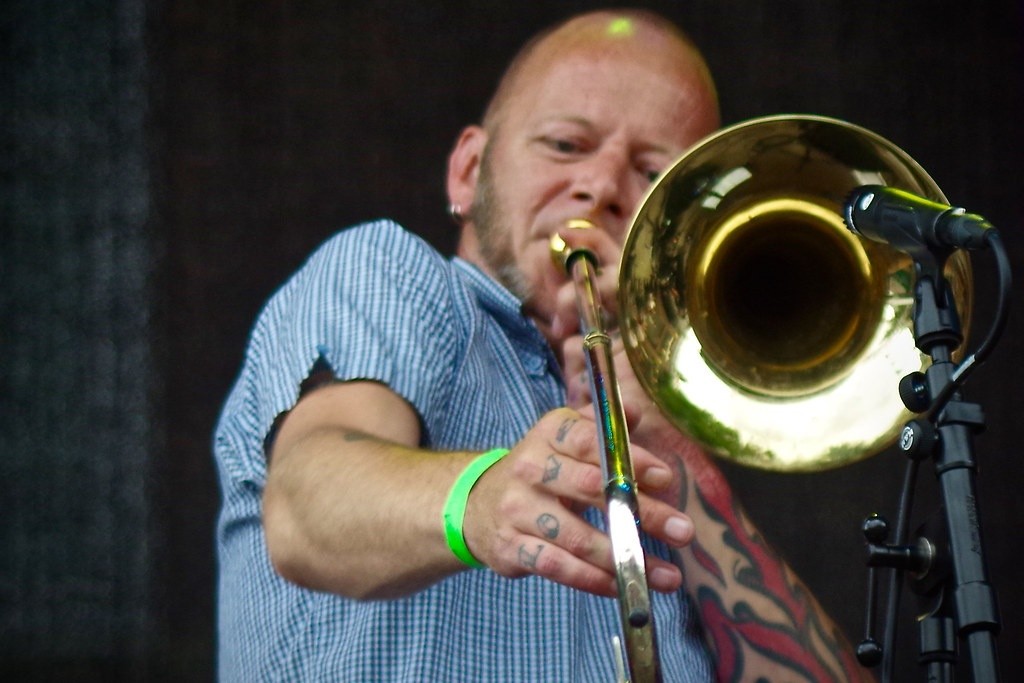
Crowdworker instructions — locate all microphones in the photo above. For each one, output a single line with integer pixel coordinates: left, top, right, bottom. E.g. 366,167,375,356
841,184,999,253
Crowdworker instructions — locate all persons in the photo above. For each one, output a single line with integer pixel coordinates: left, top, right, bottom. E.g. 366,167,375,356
211,9,724,682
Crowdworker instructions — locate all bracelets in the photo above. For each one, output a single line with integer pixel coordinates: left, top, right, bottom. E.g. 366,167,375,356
445,446,512,571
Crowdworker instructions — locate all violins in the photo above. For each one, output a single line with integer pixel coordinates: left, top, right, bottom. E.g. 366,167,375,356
548,114,979,682
